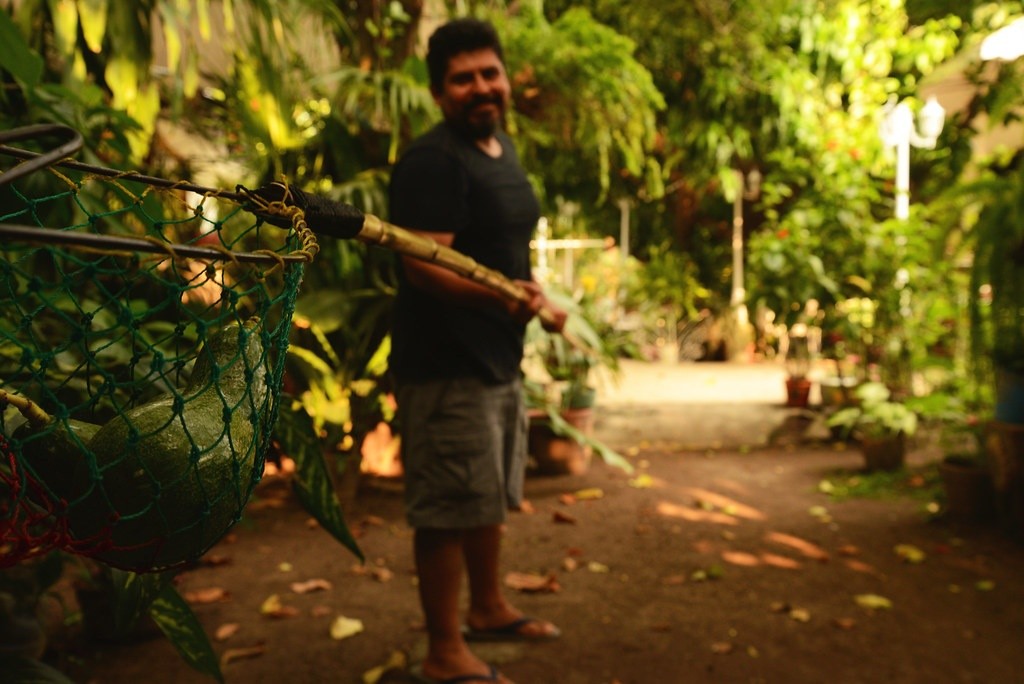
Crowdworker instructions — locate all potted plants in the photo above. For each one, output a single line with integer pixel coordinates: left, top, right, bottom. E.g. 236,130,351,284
810,384,915,472
522,268,647,480
916,199,1024,533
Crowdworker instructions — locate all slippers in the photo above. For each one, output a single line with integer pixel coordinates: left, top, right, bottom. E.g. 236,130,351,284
409,661,498,684
464,614,562,643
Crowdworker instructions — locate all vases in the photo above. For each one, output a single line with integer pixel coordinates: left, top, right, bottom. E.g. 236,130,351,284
76,574,169,645
785,375,814,410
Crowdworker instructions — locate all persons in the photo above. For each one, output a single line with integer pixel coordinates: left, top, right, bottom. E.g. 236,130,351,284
388,18,563,684
